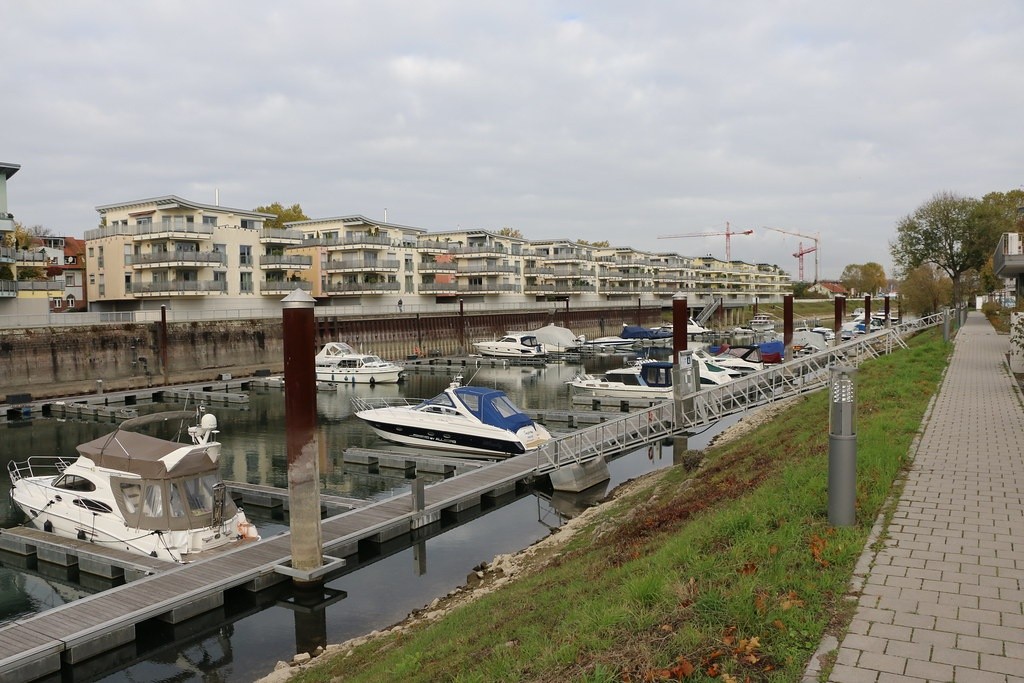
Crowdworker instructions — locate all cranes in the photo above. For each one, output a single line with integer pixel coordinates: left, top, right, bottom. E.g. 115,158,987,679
763,225,819,286
657,219,755,262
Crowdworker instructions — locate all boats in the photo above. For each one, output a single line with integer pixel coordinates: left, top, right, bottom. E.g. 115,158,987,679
470,332,551,358
562,347,786,408
565,308,901,358
353,381,554,457
315,342,404,384
8,407,263,564
